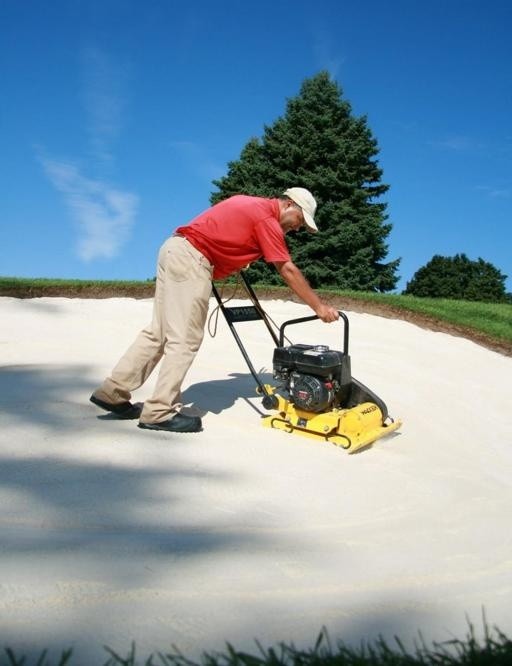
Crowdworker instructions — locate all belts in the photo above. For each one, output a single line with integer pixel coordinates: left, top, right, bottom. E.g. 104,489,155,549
172,233,186,239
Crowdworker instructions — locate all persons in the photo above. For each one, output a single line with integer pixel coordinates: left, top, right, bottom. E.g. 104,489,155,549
85,183,340,436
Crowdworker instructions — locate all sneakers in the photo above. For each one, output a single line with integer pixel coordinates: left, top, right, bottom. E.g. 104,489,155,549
138,413,200,432
90,395,142,418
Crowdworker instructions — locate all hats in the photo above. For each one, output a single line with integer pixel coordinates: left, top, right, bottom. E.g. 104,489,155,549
282,188,318,233
